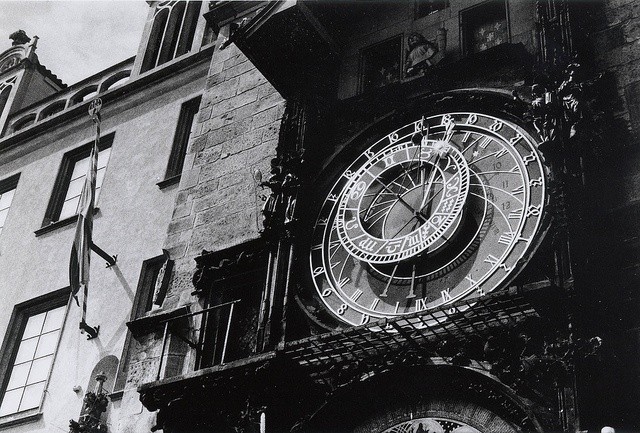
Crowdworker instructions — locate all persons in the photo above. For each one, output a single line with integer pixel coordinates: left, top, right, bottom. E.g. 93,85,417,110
406,31,437,75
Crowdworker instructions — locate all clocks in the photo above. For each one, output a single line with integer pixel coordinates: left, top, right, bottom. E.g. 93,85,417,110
290,359,543,432
286,86,560,344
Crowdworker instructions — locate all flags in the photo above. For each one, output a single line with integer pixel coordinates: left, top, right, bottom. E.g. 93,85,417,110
69,121,100,294
